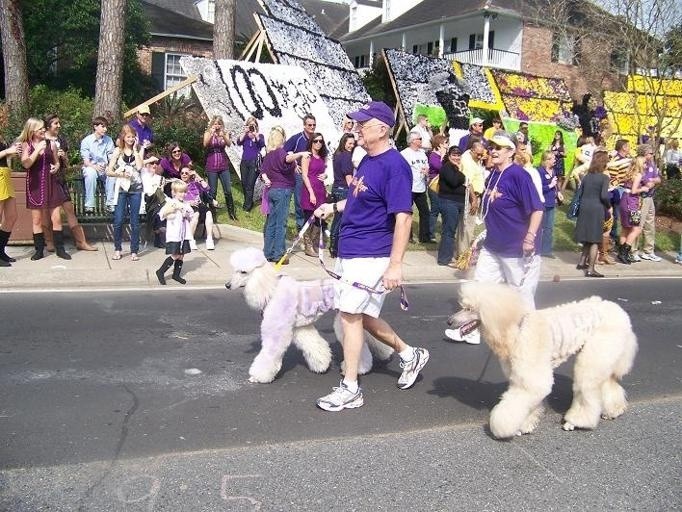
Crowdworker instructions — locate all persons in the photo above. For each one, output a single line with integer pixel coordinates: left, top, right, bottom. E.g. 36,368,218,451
80,116,115,214
436,73,473,146
571,93,612,146
47,113,98,252
0,105,23,267
12,117,71,260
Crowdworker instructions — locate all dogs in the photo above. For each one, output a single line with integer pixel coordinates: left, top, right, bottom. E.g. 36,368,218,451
444,278,641,439
224,245,397,384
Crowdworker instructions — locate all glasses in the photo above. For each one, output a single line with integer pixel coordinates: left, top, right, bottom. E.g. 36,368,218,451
487,146,511,152
356,122,384,130
312,140,323,144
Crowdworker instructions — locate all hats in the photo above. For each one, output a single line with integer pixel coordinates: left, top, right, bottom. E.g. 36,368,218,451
488,136,515,157
137,103,151,115
346,101,395,128
470,118,484,125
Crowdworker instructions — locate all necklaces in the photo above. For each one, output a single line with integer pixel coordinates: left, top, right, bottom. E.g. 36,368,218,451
26,139,46,207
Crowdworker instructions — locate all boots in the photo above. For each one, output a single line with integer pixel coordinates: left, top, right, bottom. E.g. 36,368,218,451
598,236,616,266
617,243,632,264
53,230,72,260
31,232,43,261
304,224,319,256
41,224,55,252
225,196,238,223
0,228,16,267
71,225,98,251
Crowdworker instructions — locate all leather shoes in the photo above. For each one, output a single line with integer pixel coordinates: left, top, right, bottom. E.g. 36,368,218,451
577,264,589,269
586,271,604,277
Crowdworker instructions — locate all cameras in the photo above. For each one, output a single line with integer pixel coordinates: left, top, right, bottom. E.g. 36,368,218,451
244,126,255,132
212,125,223,130
45,139,50,144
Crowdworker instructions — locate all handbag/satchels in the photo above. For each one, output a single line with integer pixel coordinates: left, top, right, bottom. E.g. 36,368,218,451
427,174,440,193
566,199,581,219
628,210,642,226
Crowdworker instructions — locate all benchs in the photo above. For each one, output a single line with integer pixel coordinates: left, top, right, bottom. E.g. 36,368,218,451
60,175,218,244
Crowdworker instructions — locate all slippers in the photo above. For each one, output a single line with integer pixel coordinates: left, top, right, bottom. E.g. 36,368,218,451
113,253,122,260
131,255,138,261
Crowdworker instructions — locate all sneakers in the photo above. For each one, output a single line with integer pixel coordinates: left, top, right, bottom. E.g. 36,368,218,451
628,252,641,262
642,252,662,261
206,239,215,250
157,270,166,285
315,379,364,412
190,240,197,250
107,206,115,214
172,275,185,284
444,327,481,346
85,207,94,213
396,347,430,390
